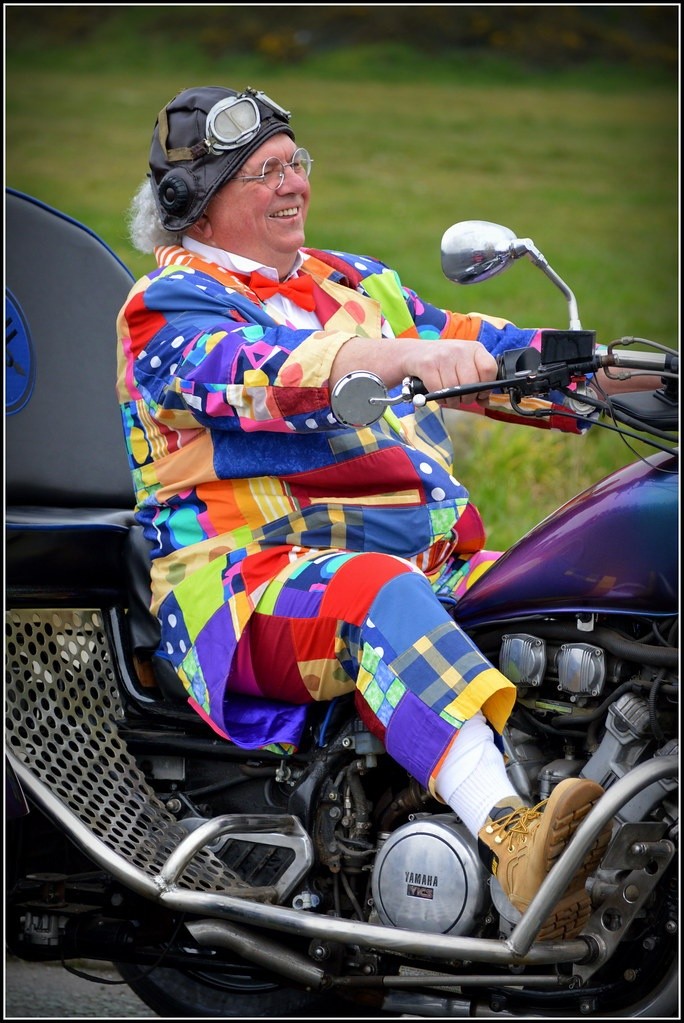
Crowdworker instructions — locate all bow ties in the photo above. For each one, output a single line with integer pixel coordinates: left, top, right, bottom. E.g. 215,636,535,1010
225,269,317,312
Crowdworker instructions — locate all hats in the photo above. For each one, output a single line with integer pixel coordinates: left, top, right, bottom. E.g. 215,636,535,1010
146,85,295,232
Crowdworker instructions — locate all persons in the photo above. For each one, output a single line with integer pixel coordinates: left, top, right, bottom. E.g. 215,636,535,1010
114,86,682,947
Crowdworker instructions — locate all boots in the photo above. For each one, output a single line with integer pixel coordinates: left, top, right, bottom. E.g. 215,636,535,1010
478,778,614,942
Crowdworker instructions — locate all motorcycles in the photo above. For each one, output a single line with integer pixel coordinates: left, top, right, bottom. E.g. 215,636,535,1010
3,181,679,1018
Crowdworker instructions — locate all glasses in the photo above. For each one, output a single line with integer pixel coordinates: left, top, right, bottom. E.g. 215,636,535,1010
205,91,291,150
229,147,314,190
